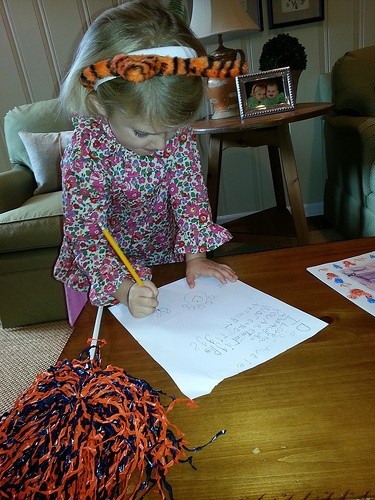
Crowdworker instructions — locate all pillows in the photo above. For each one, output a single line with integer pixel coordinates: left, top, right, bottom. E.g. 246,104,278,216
18,131,75,195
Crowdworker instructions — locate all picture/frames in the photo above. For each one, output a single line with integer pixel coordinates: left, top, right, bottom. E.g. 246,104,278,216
236,66,295,120
266,0,324,30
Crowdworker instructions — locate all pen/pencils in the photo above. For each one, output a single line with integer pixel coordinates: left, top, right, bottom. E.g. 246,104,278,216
95,219,146,287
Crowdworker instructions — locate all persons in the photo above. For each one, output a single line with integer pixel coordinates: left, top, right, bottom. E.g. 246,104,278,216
53,1,238,326
246,83,287,109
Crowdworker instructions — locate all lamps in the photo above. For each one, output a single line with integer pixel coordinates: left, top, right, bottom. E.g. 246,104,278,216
188,0,262,121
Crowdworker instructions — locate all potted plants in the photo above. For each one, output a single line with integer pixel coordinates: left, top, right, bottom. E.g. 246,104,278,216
258,33,308,105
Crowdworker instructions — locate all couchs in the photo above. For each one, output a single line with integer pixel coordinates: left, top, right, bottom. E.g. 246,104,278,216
0,97,72,330
323,46,375,237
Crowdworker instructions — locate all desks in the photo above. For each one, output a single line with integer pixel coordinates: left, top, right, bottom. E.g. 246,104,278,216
57,236,375,500
192,100,336,257
